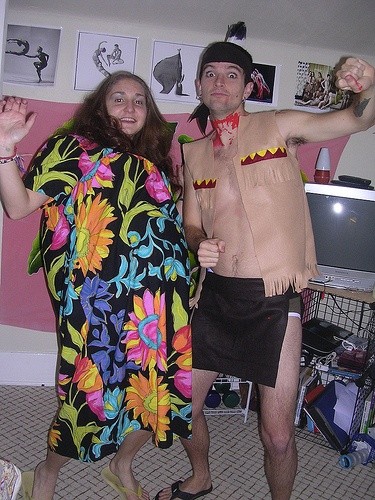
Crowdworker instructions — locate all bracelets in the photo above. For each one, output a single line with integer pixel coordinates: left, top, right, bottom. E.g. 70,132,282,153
0,145,27,176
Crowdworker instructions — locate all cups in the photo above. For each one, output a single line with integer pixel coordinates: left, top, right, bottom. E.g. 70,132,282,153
315,148,331,171
314,170,330,184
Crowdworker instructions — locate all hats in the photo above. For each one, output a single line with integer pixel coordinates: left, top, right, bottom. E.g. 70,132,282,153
201,42,254,78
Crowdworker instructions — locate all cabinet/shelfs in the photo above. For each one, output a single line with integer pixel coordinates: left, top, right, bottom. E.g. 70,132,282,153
203,282,375,458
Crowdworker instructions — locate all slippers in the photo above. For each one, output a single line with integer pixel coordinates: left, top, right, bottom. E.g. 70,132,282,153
155,480,213,500
21,470,35,500
101,466,143,500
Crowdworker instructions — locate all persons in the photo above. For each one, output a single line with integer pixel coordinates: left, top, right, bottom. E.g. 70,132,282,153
25,46,49,82
295,71,351,110
107,44,124,67
251,68,270,100
0,71,193,500
92,41,110,78
5,39,30,56
154,22,375,500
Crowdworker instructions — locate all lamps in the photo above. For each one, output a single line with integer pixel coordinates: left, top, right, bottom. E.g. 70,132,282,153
314,147,331,184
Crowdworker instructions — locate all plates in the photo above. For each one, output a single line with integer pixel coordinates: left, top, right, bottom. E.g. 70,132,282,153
338,175,371,186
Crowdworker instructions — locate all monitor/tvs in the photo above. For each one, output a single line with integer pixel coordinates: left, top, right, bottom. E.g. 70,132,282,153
304,182,375,295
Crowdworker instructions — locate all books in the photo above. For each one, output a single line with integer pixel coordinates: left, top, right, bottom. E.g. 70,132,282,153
294,367,375,453
